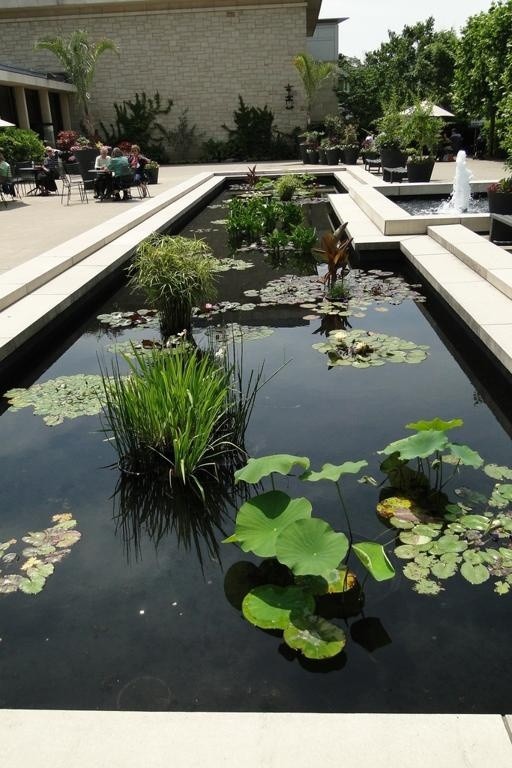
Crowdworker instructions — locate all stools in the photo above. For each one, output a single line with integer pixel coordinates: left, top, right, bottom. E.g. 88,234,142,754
383,167,408,183
489,210,512,246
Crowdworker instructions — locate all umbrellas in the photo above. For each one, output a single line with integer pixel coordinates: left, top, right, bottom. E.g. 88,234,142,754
0,119,16,126
396,99,457,117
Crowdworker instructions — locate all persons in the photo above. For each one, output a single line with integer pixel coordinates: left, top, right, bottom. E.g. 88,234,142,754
32,146,59,196
361,123,488,162
104,147,136,201
127,144,151,197
93,147,112,198
0,147,12,192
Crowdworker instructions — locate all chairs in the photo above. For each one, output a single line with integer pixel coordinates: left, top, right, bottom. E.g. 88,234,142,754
0,162,150,208
365,147,384,174
64,150,82,174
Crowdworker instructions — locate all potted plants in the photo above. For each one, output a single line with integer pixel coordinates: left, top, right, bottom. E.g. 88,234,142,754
298,120,358,165
359,147,381,164
487,174,512,213
144,161,160,184
368,85,418,171
70,136,99,180
399,88,447,182
